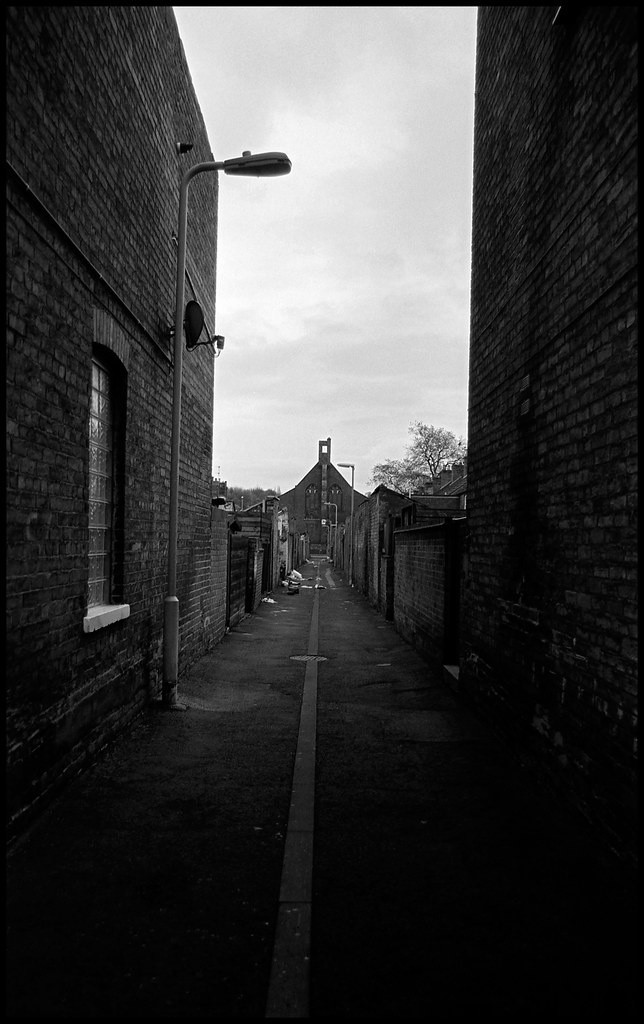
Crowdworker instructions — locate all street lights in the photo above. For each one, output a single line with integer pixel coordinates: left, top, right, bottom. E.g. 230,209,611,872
160,150,293,711
338,462,355,586
324,502,338,567
